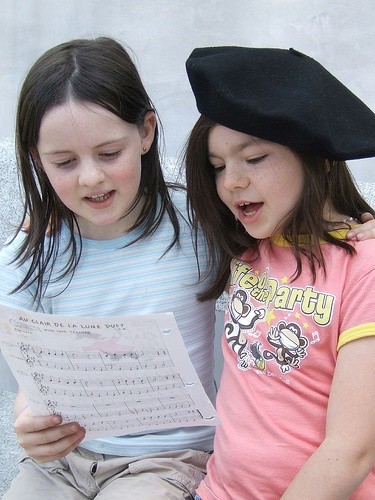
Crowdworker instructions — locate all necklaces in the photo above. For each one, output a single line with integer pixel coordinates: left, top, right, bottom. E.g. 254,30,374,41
321,213,357,230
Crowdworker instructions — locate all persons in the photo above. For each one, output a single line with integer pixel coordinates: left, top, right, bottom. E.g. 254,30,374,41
18,45,375,500
0,37,375,499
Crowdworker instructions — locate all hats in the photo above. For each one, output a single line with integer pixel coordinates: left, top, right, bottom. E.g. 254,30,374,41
185,47,374,161
